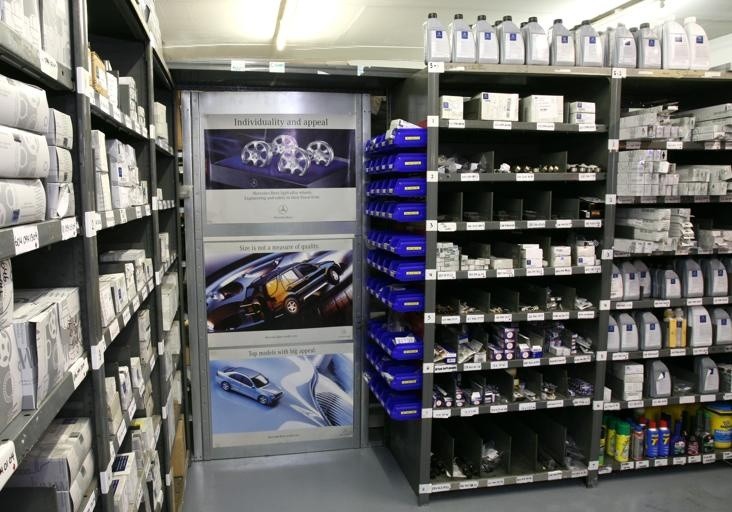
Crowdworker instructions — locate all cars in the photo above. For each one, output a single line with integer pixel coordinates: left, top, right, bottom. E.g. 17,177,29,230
217,365,285,404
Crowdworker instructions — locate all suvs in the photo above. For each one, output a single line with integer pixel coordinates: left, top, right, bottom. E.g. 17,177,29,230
240,261,342,321
206,251,334,332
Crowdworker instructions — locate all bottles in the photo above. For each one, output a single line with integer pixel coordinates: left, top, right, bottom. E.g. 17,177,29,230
599,410,717,466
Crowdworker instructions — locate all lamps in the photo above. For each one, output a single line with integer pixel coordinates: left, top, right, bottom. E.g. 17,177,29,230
272,0,296,50
567,0,663,30
